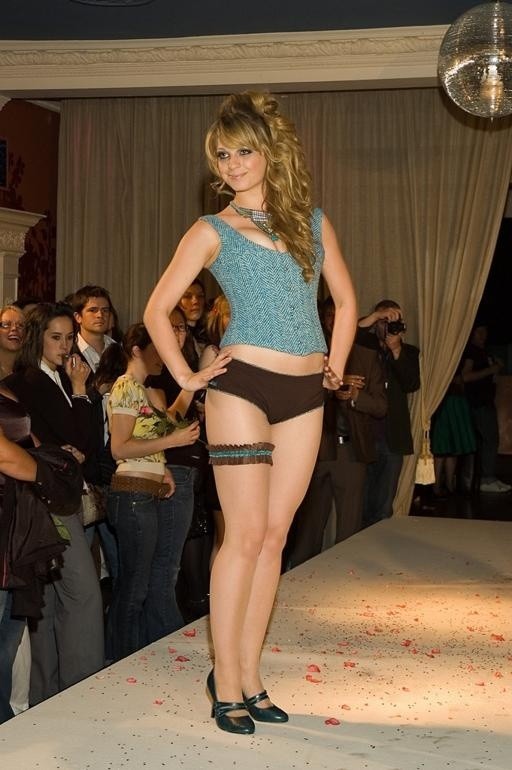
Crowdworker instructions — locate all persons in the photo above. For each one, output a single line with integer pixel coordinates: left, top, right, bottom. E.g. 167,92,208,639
142,92,360,735
100,323,202,661
415,322,505,512
286,294,421,570
1,279,230,724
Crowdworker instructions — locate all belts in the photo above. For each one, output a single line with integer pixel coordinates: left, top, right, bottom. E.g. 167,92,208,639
335,434,350,445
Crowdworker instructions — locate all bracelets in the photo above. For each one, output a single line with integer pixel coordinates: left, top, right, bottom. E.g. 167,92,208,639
322,356,344,386
72,394,92,403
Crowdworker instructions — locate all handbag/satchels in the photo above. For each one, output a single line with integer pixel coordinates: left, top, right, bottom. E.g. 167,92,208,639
80,486,108,528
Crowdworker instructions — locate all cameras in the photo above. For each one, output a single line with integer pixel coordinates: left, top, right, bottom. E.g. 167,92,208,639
384,314,407,336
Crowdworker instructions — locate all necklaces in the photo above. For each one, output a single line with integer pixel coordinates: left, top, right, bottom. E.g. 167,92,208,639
229,197,283,241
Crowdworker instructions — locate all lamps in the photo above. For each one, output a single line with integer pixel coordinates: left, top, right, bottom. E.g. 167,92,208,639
435,2,512,125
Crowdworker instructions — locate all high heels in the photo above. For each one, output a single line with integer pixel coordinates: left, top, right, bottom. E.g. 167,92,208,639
242,686,289,723
207,666,254,733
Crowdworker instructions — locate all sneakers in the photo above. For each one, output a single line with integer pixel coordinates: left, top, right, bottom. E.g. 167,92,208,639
480,478,511,492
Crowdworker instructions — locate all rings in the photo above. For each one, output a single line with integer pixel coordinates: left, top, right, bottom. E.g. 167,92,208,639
65,447,73,453
82,364,85,366
